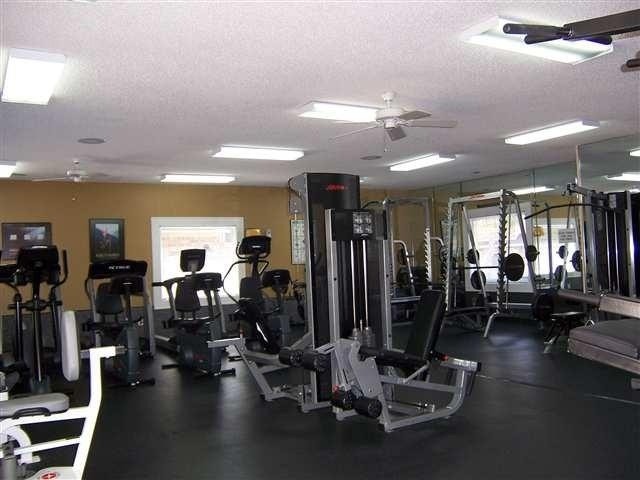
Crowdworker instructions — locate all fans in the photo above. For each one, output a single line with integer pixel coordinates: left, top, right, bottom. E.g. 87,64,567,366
330,85,433,149
32,158,123,187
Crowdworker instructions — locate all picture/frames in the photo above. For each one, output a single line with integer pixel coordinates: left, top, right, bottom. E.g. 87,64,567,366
1,222,52,261
88,217,126,263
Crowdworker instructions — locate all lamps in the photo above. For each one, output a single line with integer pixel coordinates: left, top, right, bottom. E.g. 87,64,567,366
503,118,603,147
388,152,458,173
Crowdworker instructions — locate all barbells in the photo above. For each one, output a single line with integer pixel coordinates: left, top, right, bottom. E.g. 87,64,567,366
441,254,524,281
